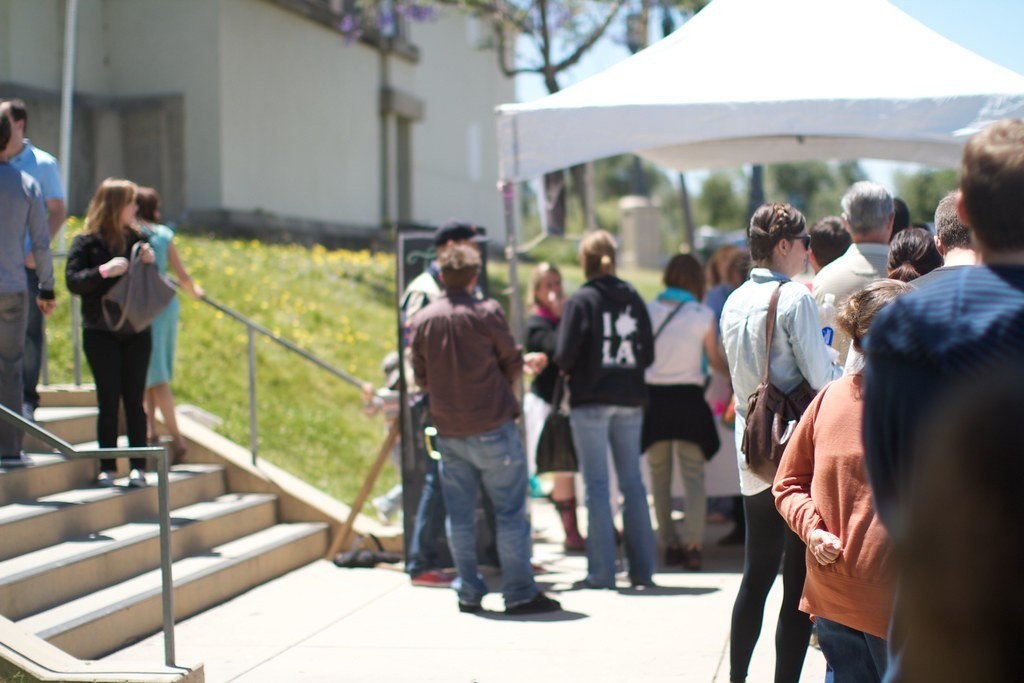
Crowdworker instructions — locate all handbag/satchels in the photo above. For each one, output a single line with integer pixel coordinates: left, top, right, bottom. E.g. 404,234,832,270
102,240,177,332
536,374,580,476
741,379,819,483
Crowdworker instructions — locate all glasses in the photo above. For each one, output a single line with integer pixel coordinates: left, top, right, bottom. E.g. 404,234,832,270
787,235,812,252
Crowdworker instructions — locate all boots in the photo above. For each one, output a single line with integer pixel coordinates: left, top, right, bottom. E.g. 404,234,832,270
548,494,586,551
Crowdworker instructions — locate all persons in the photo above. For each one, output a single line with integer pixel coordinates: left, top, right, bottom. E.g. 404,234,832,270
0,97,66,467
131,187,204,465
400,120,1024,682
65,177,156,489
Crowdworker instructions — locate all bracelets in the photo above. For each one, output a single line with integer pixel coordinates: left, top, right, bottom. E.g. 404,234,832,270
98,264,107,278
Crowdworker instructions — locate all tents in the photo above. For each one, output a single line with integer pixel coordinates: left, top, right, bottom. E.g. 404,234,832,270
495,1,1024,459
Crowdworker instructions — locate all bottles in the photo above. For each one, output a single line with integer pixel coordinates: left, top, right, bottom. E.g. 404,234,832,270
818,293,838,350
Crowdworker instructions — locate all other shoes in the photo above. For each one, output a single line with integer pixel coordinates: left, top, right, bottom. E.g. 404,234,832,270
0,455,34,466
658,542,703,571
22,405,35,422
128,469,147,487
573,577,592,589
412,569,458,587
717,529,745,547
458,601,481,612
96,472,113,486
505,590,561,615
